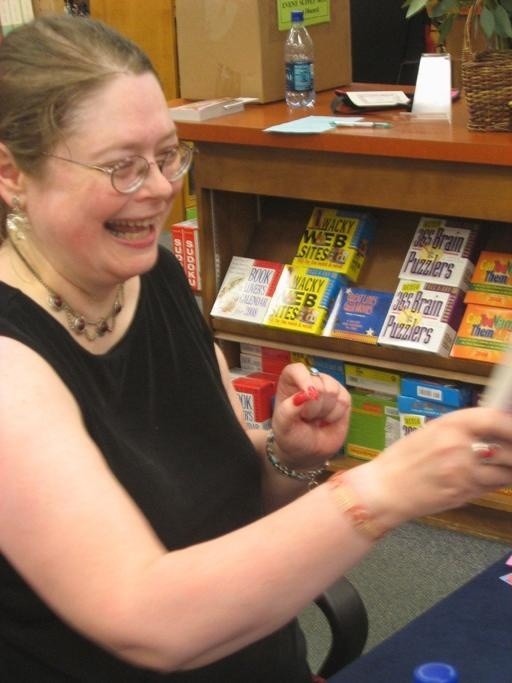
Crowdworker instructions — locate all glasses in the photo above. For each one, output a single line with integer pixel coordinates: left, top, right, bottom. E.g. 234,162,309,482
37,137,201,196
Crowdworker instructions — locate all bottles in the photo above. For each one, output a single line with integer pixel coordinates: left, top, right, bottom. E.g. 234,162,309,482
281,9,317,107
412,662,460,682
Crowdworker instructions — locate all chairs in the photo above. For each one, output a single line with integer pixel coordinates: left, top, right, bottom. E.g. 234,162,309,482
310,574,370,681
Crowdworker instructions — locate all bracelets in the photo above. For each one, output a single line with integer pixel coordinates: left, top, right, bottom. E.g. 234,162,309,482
263,432,329,487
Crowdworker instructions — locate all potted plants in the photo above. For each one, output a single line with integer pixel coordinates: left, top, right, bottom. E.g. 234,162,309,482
398,0,512,133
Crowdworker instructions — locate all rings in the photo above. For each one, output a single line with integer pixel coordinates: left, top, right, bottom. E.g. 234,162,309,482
472,439,501,464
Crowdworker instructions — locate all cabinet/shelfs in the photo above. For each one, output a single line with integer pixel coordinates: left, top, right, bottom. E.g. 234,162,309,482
164,98,512,546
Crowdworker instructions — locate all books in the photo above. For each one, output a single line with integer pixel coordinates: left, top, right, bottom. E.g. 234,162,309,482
173,96,245,122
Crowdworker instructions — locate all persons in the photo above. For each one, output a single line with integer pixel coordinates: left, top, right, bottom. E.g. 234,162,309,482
0,15,509,683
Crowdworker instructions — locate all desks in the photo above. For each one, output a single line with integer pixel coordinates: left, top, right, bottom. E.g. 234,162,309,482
327,548,512,682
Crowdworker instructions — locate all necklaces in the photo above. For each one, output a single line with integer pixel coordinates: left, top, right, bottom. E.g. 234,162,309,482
7,229,131,342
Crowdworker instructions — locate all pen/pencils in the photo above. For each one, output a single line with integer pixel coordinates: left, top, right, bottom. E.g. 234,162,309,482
328,120,392,128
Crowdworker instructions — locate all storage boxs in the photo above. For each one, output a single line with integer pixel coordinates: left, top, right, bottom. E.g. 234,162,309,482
173,0,354,107
